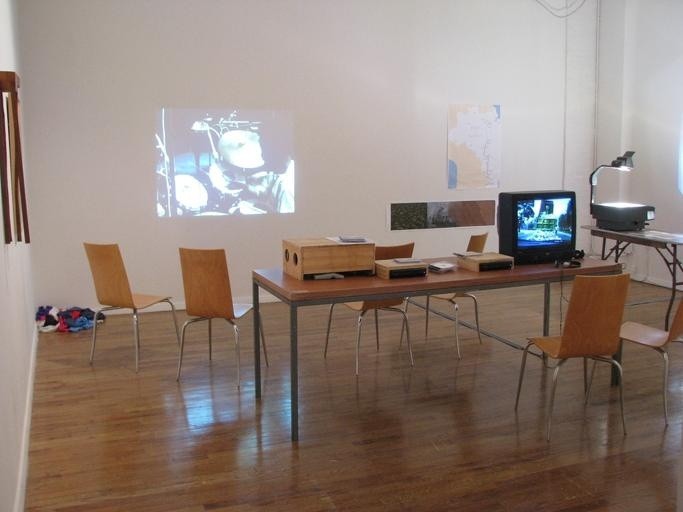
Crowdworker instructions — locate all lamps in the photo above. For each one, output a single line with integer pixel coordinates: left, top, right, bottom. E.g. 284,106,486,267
398,233,489,360
514,272,631,443
175,247,269,390
583,292,683,427
325,242,416,376
83,242,181,374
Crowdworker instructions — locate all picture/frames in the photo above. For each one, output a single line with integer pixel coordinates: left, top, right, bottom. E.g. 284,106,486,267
252,255,622,443
586,227,683,331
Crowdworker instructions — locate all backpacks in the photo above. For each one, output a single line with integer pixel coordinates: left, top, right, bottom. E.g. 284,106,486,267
208,130,264,195
174,174,208,212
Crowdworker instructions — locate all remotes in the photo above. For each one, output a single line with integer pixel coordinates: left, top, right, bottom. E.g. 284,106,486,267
563,261,580,267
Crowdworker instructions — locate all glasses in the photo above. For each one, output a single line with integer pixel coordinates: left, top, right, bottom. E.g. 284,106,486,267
589,150,655,232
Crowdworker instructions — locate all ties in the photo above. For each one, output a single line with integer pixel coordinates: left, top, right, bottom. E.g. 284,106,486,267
499,190,576,264
